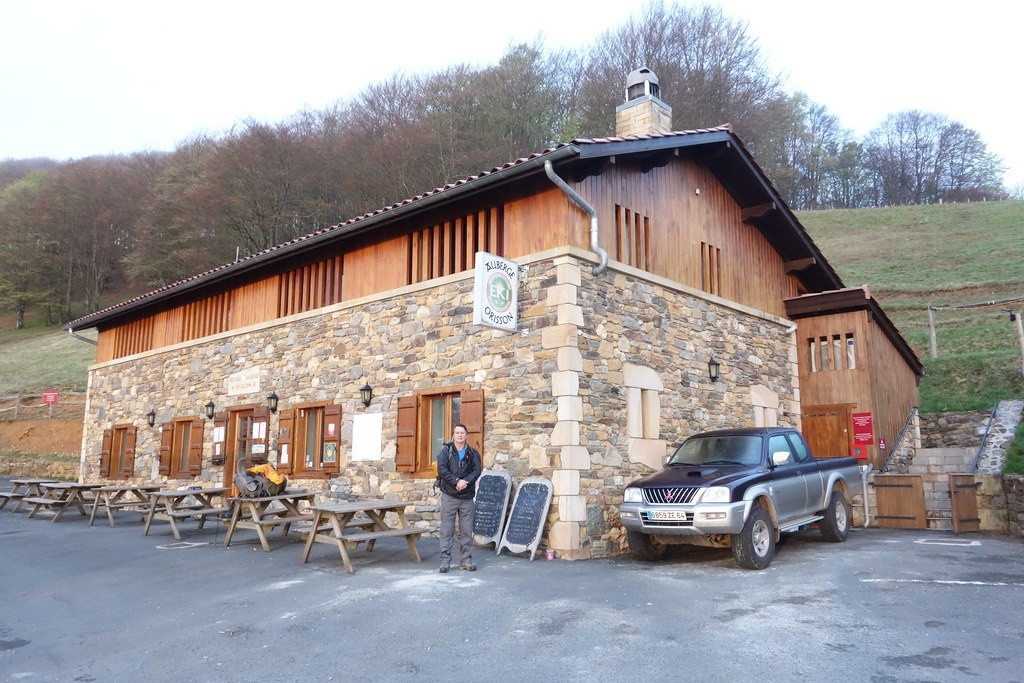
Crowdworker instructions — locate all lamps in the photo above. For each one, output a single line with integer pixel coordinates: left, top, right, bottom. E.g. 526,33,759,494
267,390,278,413
205,399,215,419
709,357,720,382
359,382,372,407
146,409,155,426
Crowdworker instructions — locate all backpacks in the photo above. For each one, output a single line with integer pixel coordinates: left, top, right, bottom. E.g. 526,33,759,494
434,440,454,487
231,456,287,498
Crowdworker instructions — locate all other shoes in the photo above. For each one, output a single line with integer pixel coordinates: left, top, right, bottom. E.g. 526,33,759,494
439,562,450,572
460,558,476,571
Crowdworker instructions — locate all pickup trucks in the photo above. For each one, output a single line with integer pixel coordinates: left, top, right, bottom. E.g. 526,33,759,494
619,425,862,571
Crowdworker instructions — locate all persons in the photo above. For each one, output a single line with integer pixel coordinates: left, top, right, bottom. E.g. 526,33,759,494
437,423,481,572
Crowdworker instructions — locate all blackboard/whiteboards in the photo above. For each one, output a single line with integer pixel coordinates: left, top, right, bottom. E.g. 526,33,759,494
472,474,507,537
505,483,549,546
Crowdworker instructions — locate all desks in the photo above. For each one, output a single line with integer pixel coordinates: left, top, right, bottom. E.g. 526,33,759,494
89,485,167,527
141,488,231,539
299,499,421,574
28,483,107,522
222,491,326,551
0,479,62,513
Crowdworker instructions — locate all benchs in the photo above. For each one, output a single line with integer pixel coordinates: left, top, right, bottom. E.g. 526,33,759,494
168,507,230,519
253,515,314,525
291,521,374,535
0,492,167,509
136,505,204,514
218,511,284,522
337,526,429,542
